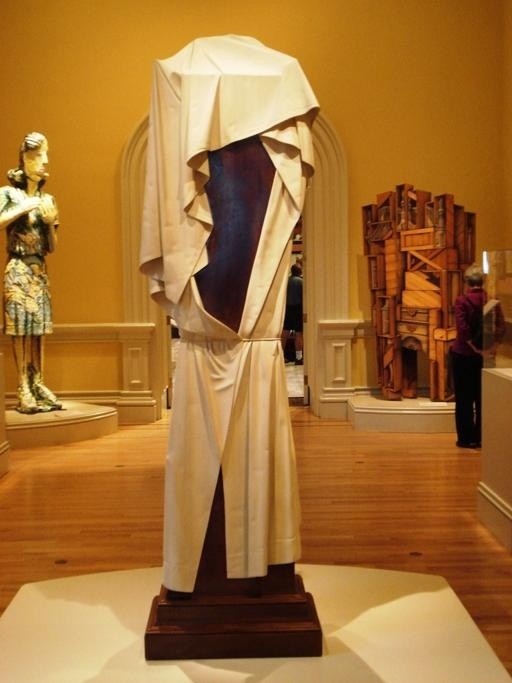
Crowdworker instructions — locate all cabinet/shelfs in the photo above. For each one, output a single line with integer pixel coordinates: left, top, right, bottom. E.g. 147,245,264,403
473,249,511,546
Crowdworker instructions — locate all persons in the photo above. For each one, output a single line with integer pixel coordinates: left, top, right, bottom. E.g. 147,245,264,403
1,128,64,418
445,266,507,451
286,261,304,305
